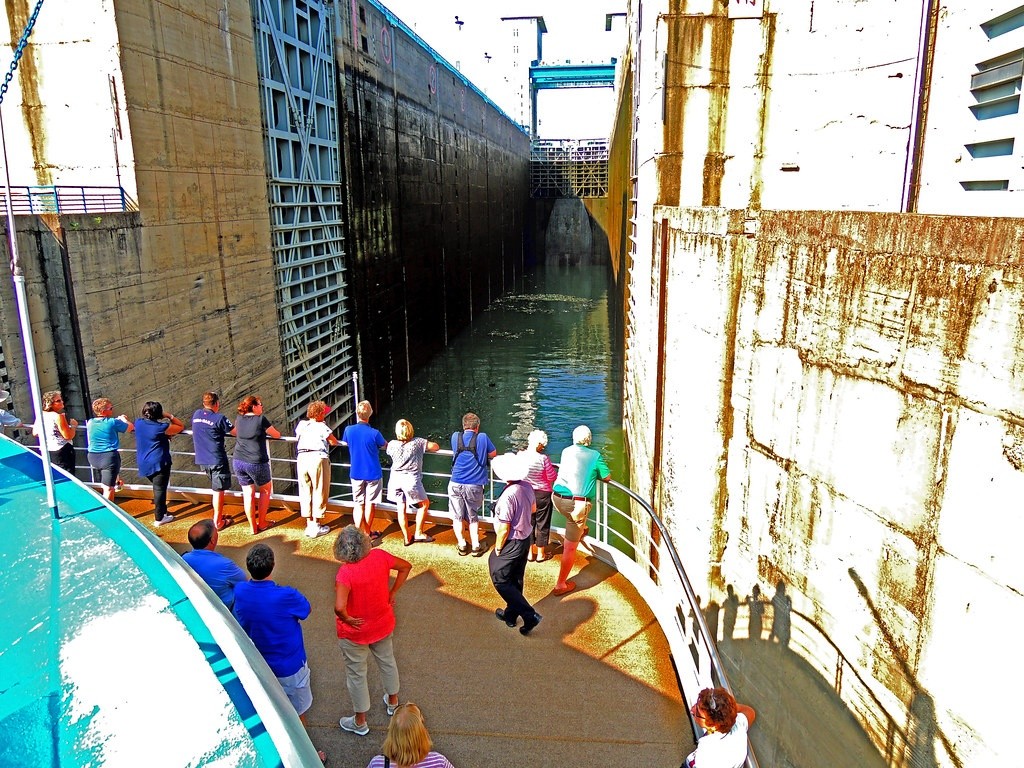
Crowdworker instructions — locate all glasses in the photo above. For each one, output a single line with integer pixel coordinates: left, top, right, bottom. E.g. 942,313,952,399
105,407,113,411
54,399,61,403
694,702,706,720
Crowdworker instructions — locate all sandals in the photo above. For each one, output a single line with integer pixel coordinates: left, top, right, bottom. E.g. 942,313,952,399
458,541,472,556
471,542,488,557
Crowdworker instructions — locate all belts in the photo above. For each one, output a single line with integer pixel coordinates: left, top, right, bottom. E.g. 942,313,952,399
554,492,591,501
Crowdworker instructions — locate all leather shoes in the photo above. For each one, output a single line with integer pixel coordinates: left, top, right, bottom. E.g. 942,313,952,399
496,608,518,627
520,613,542,635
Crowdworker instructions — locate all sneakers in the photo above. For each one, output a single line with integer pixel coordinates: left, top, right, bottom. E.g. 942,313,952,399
382,694,398,715
155,514,173,526
304,526,311,535
340,716,370,735
310,522,330,538
114,479,124,493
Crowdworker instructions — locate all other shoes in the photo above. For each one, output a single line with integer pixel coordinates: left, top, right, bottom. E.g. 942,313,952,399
554,582,576,596
580,525,590,540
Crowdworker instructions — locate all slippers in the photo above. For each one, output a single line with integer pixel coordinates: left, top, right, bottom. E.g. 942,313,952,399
527,558,535,562
414,534,436,542
405,535,414,546
261,521,275,530
369,532,382,540
537,553,553,562
219,519,234,531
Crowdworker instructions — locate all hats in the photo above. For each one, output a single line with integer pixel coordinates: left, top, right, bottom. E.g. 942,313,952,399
491,453,528,481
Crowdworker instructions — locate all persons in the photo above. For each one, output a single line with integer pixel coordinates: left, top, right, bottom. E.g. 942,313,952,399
342,401,388,540
295,401,338,538
550,425,611,596
487,453,543,636
386,419,439,546
334,525,412,736
180,519,250,611
86,398,135,503
517,430,558,563
134,401,185,527
367,701,455,768
681,688,755,768
231,544,327,764
230,396,280,535
447,413,496,558
33,391,78,475
0,390,23,434
191,392,236,532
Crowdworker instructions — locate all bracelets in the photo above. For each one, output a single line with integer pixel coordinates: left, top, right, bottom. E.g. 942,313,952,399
71,425,77,428
495,548,501,551
168,414,173,420
